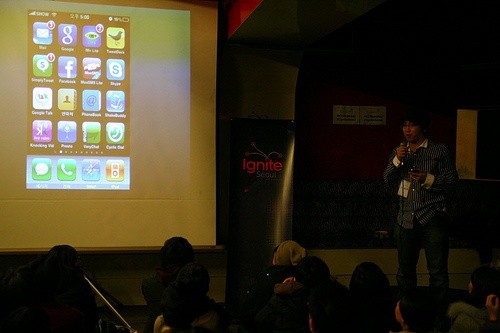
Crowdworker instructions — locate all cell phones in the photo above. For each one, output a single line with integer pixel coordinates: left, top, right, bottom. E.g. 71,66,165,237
409,169,420,173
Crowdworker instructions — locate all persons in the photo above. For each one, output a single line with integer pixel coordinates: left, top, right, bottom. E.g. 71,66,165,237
0,237,500,333
383,110,459,326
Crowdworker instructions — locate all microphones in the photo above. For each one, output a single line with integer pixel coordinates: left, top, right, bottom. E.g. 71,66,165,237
400,142,406,166
